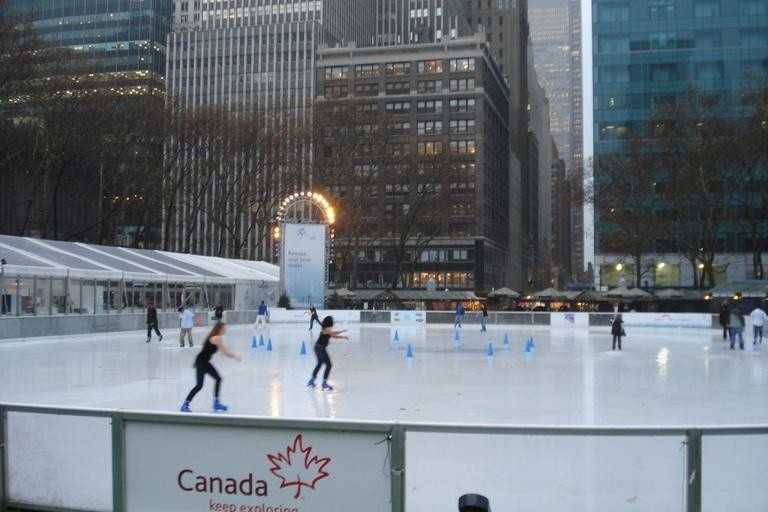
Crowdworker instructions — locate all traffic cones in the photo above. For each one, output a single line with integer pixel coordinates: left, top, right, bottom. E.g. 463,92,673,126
525,340,531,353
405,343,414,358
258,333,264,345
266,338,273,351
486,342,494,356
251,336,257,348
502,332,511,344
455,327,460,341
299,340,309,355
529,336,535,348
393,329,399,341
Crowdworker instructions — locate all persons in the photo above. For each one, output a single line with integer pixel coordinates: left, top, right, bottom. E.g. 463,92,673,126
751,305,768,345
253,299,270,329
727,309,746,349
304,301,322,331
145,300,163,342
181,321,241,411
452,303,466,328
211,303,222,321
308,315,348,389
178,305,193,347
475,304,488,331
720,304,731,338
612,314,625,351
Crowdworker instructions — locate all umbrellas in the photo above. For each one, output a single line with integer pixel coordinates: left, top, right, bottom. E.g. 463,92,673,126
488,286,686,311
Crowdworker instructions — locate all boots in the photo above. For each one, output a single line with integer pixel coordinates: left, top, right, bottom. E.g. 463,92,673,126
321,380,332,387
181,400,192,412
308,379,315,385
213,399,226,409
